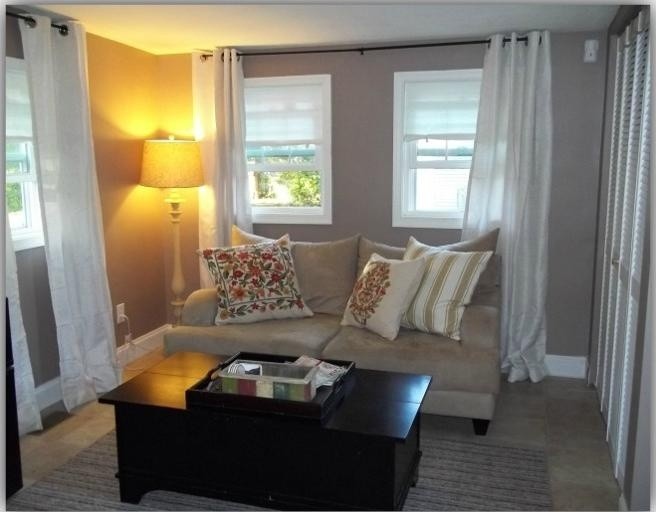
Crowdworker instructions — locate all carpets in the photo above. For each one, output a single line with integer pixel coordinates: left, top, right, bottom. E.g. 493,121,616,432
5,429,557,512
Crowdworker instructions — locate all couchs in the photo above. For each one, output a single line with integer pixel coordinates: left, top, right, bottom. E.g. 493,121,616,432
163,225,509,435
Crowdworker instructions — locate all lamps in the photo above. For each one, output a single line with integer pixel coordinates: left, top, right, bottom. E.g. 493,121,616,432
141,139,205,327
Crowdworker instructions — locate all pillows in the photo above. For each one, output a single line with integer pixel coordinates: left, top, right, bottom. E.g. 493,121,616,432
196,231,493,342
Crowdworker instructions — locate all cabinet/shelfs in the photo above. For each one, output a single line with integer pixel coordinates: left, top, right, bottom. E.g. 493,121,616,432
589,4,656,484
5,296,25,496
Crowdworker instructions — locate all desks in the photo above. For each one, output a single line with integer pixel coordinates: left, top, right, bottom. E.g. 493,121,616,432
99,348,434,511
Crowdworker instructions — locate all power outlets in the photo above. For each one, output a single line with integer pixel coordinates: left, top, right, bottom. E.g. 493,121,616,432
114,302,127,323
